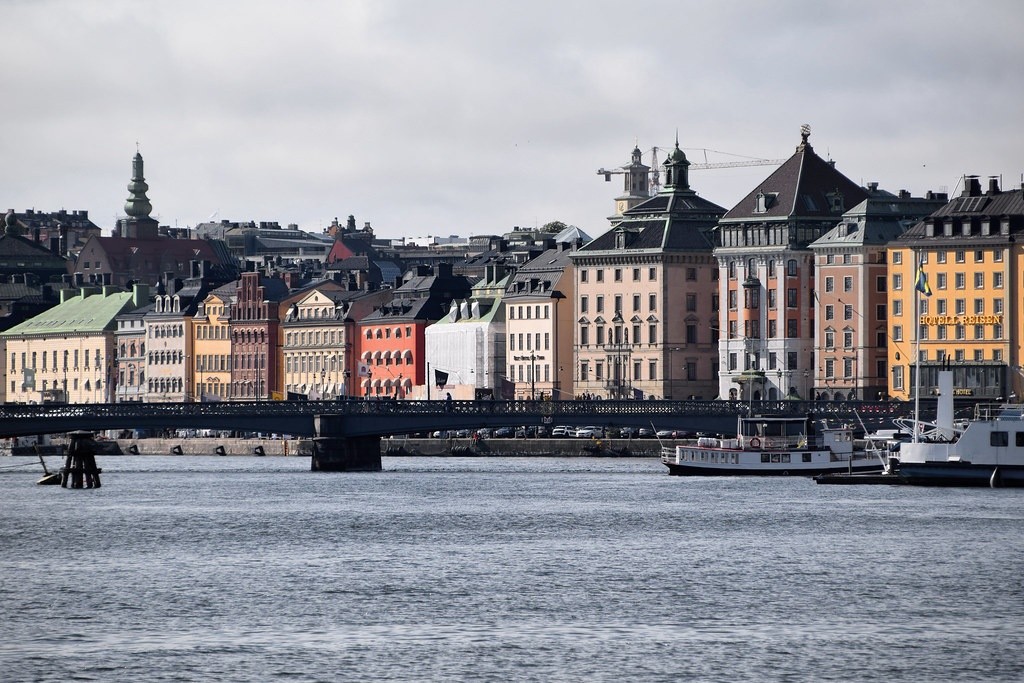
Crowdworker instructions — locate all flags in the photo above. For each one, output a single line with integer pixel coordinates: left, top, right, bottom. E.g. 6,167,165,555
23,368,35,389
272,390,284,401
202,392,221,402
360,326,412,387
358,361,369,376
914,266,932,297
435,369,448,387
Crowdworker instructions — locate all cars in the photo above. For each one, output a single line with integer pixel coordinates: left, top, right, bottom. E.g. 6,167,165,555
156,427,691,439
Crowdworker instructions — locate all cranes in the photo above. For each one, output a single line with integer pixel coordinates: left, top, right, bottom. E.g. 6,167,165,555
592,144,786,197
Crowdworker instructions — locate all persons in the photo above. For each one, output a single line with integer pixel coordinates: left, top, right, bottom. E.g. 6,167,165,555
582,393,591,401
815,392,821,412
446,392,452,411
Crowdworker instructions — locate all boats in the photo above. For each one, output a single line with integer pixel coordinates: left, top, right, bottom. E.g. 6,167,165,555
653,415,896,475
886,402,1024,483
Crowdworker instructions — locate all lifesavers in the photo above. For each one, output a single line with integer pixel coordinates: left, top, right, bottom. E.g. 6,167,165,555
749,437,761,448
